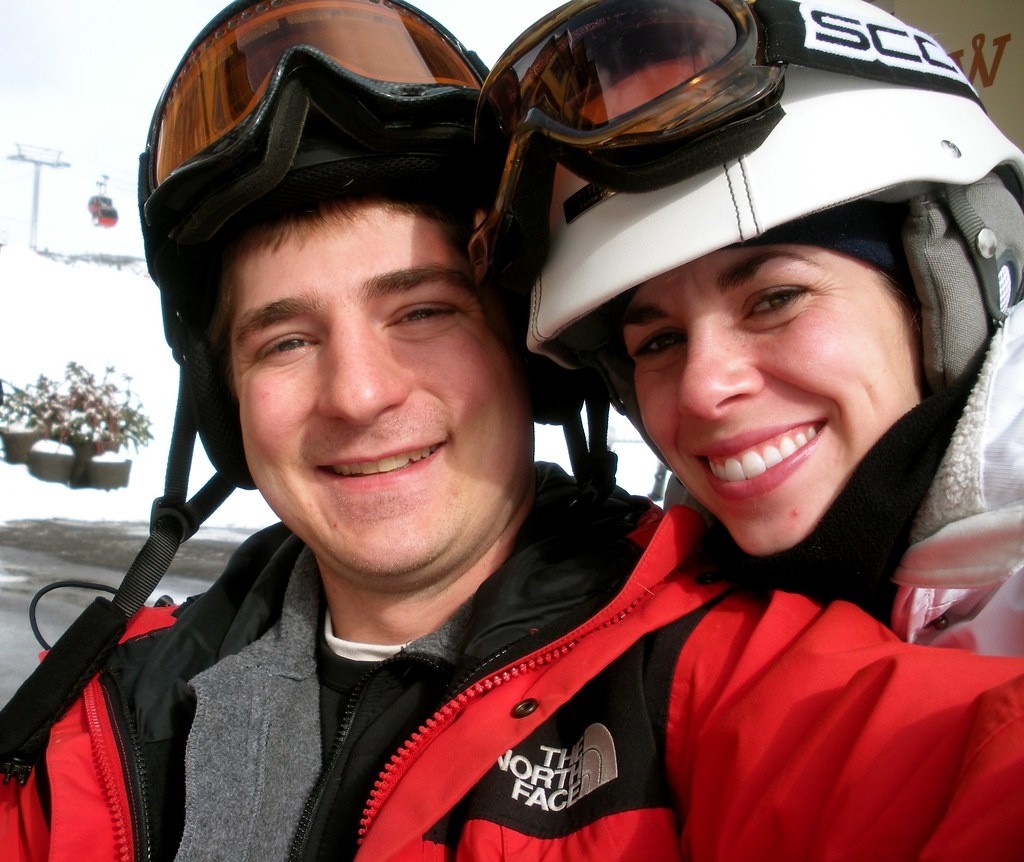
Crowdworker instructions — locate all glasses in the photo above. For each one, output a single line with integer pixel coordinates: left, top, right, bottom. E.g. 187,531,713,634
468,0,987,333
134,0,493,345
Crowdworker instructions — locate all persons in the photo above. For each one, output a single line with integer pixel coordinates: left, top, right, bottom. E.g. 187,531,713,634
0,0,1024,862
471,0,1024,733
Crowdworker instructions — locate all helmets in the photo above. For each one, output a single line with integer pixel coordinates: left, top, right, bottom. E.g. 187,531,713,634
524,0,1024,375
